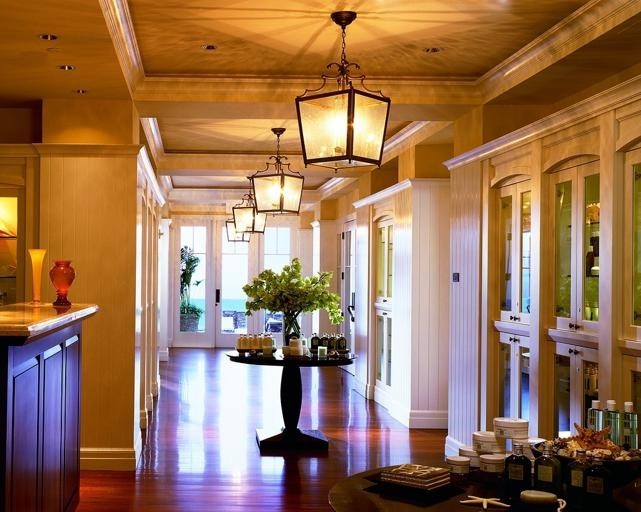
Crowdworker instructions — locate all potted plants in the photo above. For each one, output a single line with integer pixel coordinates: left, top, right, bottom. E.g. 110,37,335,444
180,246,204,331
242,257,344,345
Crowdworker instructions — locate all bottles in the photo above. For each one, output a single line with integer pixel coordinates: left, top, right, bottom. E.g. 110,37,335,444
299,331,346,359
585,202,599,258
584,300,591,320
584,245,594,275
504,399,638,512
235,331,275,356
583,364,599,398
592,302,598,321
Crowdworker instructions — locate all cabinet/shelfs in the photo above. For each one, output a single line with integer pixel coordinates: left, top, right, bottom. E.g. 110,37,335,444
442,75,641,464
352,178,450,429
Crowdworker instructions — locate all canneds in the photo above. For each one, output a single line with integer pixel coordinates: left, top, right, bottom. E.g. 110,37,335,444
447,417,546,486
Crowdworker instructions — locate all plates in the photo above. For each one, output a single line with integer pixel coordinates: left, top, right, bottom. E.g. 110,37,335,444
360,472,471,498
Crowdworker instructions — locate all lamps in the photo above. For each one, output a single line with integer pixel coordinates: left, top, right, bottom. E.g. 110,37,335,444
226,218,251,243
233,177,267,235
296,11,390,173
251,128,304,217
